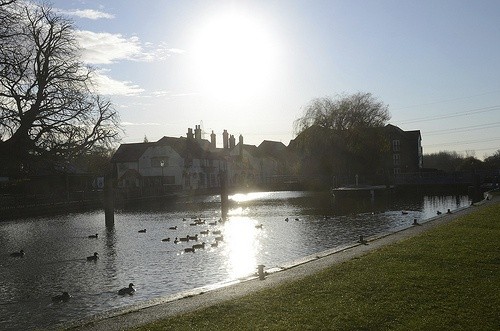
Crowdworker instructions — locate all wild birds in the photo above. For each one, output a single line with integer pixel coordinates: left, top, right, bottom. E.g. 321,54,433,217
11,209,443,303
